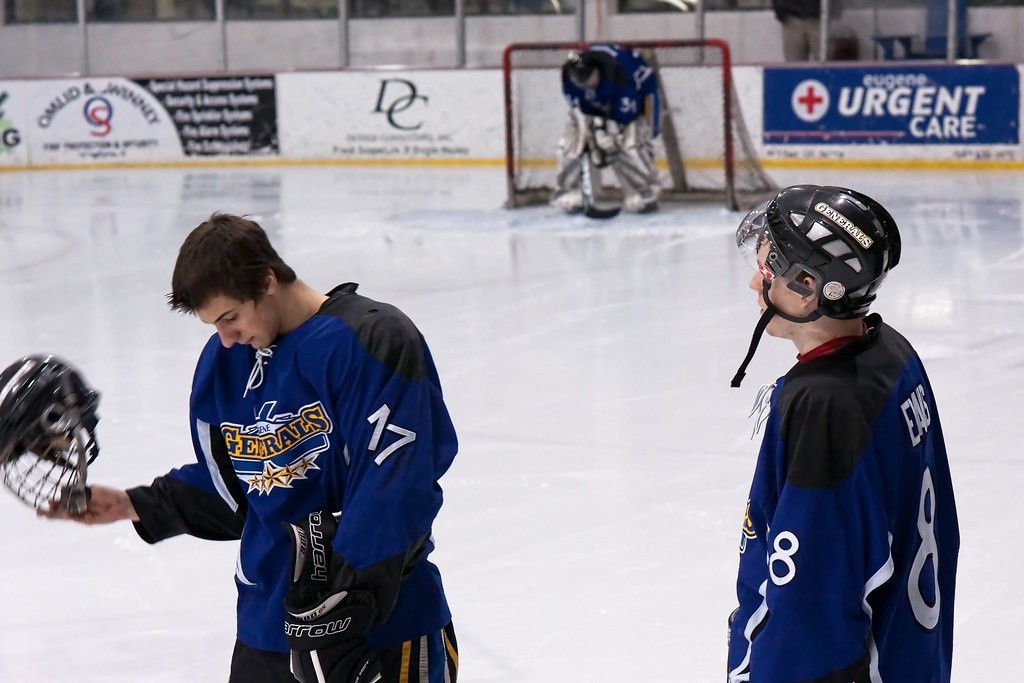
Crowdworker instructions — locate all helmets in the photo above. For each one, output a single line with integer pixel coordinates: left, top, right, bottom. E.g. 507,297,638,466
736,184,901,320
567,50,598,85
0,353,102,514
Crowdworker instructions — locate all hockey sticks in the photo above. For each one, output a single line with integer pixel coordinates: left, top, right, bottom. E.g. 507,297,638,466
582,146,621,219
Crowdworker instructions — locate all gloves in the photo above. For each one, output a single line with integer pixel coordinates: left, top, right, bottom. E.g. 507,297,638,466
281,509,382,683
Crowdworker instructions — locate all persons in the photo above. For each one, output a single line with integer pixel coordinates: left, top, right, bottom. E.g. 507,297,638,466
730,183,961,682
37,214,461,683
553,43,665,219
